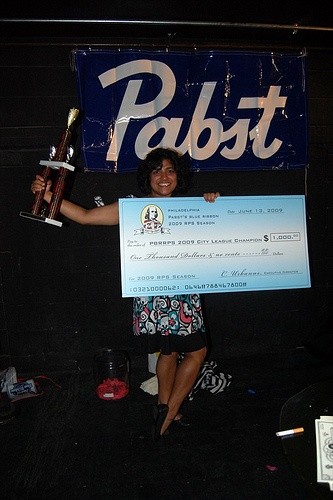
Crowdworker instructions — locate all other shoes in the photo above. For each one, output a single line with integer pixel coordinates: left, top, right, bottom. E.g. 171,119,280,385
155,404,191,427
152,435,171,463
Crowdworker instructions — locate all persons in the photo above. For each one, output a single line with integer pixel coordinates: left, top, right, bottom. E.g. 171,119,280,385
31,147,222,441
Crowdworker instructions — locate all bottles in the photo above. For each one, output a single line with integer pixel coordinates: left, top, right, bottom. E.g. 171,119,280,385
92,344,129,400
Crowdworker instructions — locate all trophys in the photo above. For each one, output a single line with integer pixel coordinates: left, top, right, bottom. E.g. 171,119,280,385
20,107,78,228
